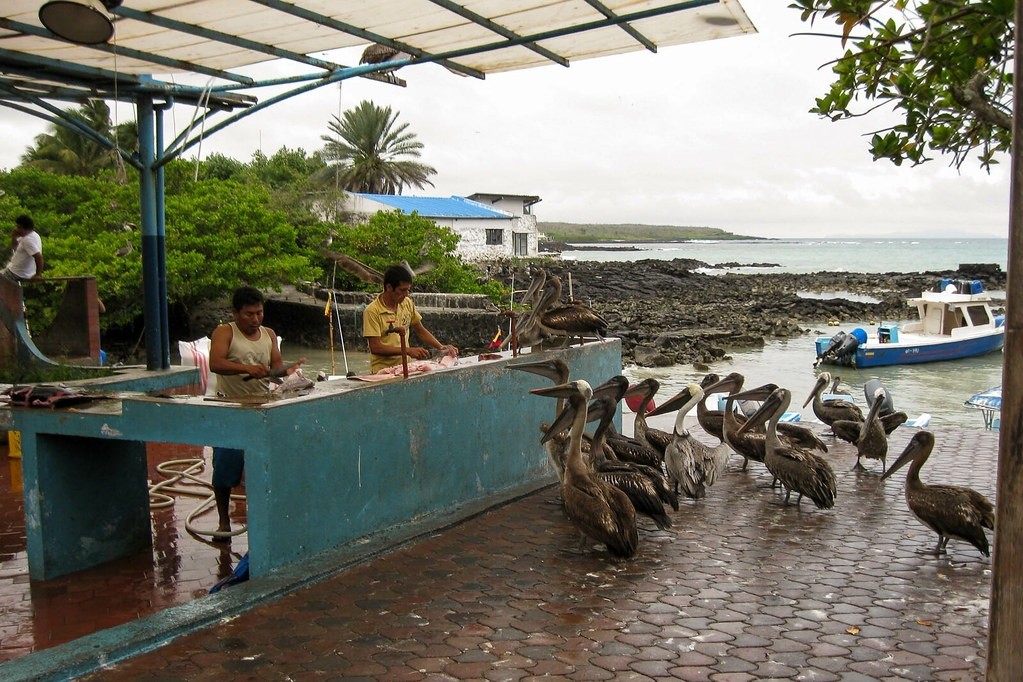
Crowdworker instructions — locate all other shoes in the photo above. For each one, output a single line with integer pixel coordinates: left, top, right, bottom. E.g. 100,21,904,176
212,528,231,541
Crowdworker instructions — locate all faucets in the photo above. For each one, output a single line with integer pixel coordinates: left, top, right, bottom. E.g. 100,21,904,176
384,320,396,336
496,304,506,319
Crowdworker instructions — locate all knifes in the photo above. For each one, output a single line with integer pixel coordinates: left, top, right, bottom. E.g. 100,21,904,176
429,348,440,356
243,366,292,381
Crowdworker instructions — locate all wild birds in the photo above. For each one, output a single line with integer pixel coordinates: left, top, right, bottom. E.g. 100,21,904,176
501,358,910,561
319,229,436,288
879,430,996,558
497,269,609,355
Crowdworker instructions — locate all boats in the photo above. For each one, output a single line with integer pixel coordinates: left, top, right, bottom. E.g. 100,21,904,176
813,278,1006,371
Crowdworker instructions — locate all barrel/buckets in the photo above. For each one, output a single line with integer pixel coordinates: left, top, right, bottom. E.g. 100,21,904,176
995,313,1006,327
940,278,983,294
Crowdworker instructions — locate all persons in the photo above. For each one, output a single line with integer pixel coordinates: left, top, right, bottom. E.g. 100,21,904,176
209,288,305,542
362,265,458,376
0,215,43,312
